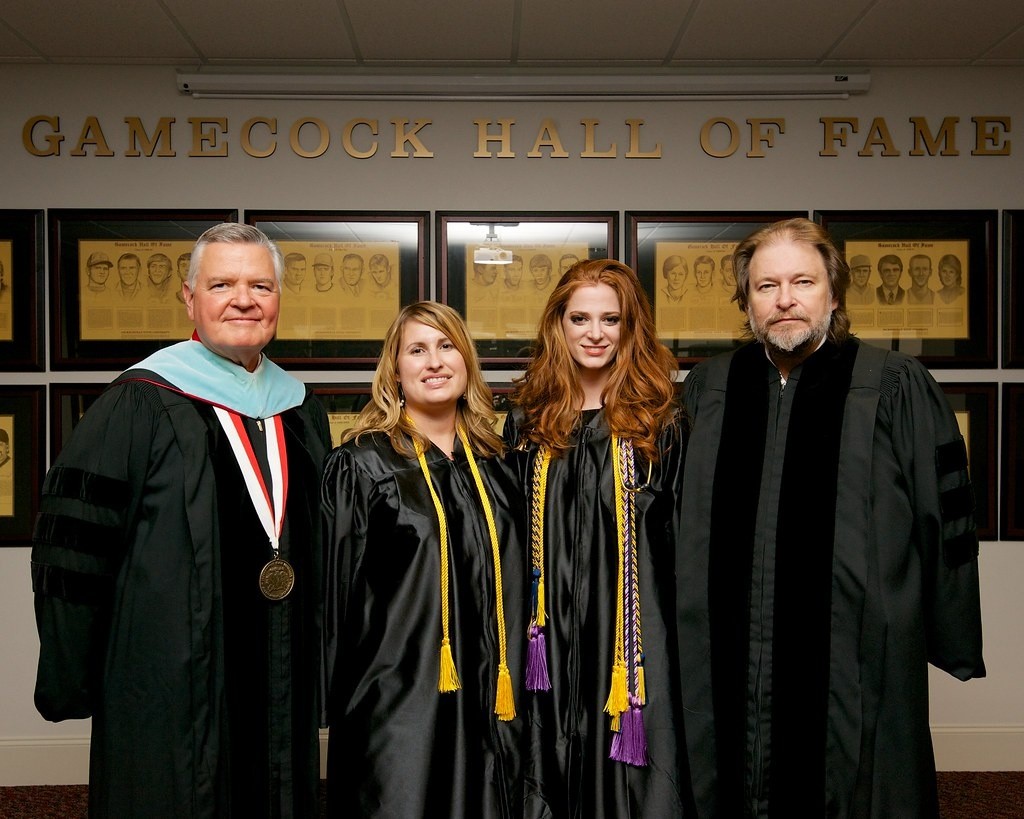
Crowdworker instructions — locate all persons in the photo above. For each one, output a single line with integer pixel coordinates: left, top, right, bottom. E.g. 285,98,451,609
501,257,702,819
663,217,992,819
33,218,339,819
311,298,535,818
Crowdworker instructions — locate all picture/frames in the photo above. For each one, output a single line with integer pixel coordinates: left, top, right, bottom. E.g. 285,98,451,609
1000,383,1024,542
50,383,113,471
45,206,239,372
470,382,531,444
622,209,812,369
0,384,47,548
434,210,622,369
1001,207,1023,371
934,381,997,543
242,206,432,372
299,382,393,453
814,210,996,361
0,207,43,375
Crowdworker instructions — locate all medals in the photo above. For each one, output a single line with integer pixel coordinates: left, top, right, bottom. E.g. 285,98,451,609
259,552,297,601
